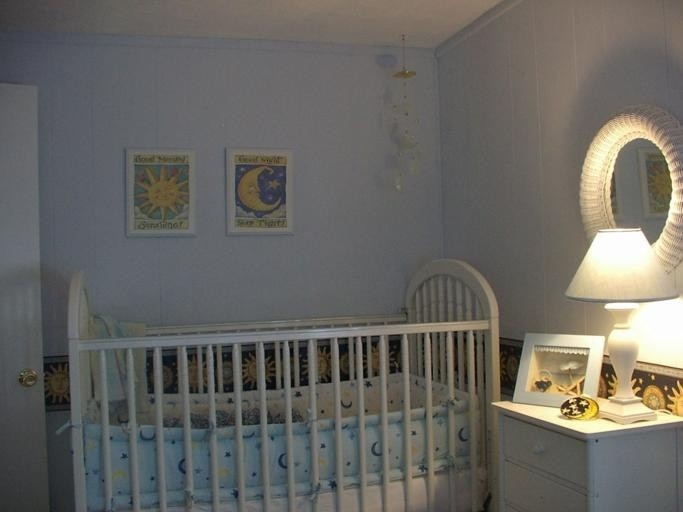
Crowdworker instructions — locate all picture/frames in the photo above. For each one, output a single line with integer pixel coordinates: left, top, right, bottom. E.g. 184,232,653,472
610,160,624,220
637,146,672,220
125,146,197,238
224,146,295,236
512,333,606,408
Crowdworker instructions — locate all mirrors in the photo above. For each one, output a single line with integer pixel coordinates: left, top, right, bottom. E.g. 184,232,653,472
578,106,682,276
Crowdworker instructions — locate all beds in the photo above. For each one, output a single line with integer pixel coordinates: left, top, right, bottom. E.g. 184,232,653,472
67,261,501,512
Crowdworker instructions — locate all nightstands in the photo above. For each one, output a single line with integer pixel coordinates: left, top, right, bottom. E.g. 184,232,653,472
491,400,683,512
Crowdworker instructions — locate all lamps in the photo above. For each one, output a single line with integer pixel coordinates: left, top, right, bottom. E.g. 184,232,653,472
565,228,679,426
